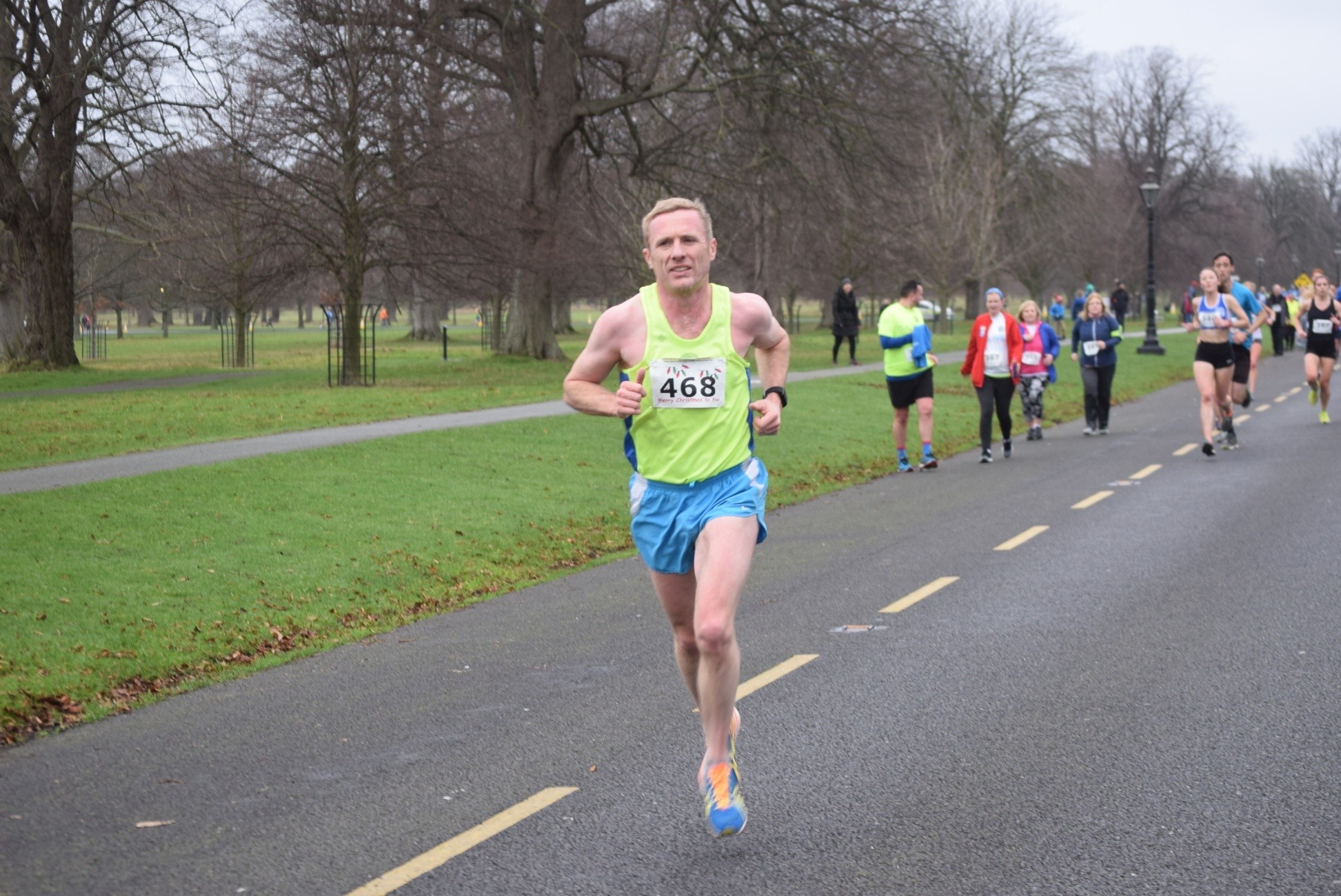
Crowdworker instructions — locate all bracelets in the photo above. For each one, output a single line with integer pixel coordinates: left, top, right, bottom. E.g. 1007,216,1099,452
1271,317,1276,321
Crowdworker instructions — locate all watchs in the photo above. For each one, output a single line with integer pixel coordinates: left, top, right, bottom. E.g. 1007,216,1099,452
1226,319,1232,328
762,386,788,407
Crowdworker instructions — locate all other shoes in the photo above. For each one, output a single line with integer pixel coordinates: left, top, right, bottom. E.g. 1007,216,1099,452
832,361,837,364
850,360,861,366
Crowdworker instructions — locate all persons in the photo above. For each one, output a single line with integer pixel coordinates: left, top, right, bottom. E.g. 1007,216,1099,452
82,314,91,334
379,306,389,328
563,195,791,839
880,298,890,313
326,306,333,328
1071,291,1124,437
959,288,1024,463
1049,294,1066,339
1180,252,1276,456
264,313,275,332
831,277,862,366
1071,277,1129,334
1181,267,1341,424
878,278,939,474
1016,299,1060,441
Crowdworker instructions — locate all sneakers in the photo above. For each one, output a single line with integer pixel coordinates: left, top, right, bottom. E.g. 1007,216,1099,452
1309,382,1320,405
897,460,914,473
697,754,749,839
1221,434,1237,450
919,455,937,469
1003,439,1012,459
1241,389,1251,409
728,708,741,756
1083,427,1093,436
1202,443,1217,457
1216,433,1224,443
1222,409,1235,433
981,449,993,463
1100,430,1109,436
1319,411,1331,424
1027,429,1033,440
1034,427,1042,440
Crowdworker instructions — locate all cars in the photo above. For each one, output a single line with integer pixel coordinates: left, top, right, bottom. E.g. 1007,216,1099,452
916,299,953,322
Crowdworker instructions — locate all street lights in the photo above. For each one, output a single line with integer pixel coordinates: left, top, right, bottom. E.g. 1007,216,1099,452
1255,252,1266,296
1136,165,1167,355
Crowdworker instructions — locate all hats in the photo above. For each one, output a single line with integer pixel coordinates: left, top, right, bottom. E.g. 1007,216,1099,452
1086,284,1095,291
986,288,1006,302
841,278,850,284
1055,294,1063,302
